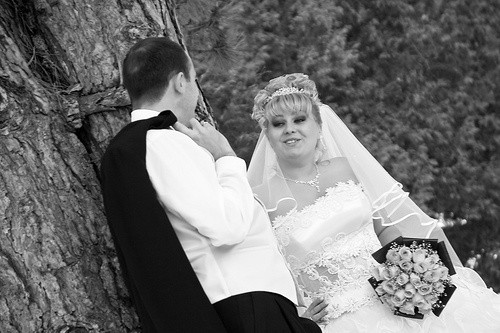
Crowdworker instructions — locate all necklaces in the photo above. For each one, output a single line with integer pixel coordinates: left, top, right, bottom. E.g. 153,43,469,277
280,160,325,193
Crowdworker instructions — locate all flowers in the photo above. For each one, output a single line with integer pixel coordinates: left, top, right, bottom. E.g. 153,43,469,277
372,239,450,315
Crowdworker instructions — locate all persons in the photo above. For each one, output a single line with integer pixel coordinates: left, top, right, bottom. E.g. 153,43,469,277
98,37,321,333
241,72,499,333
